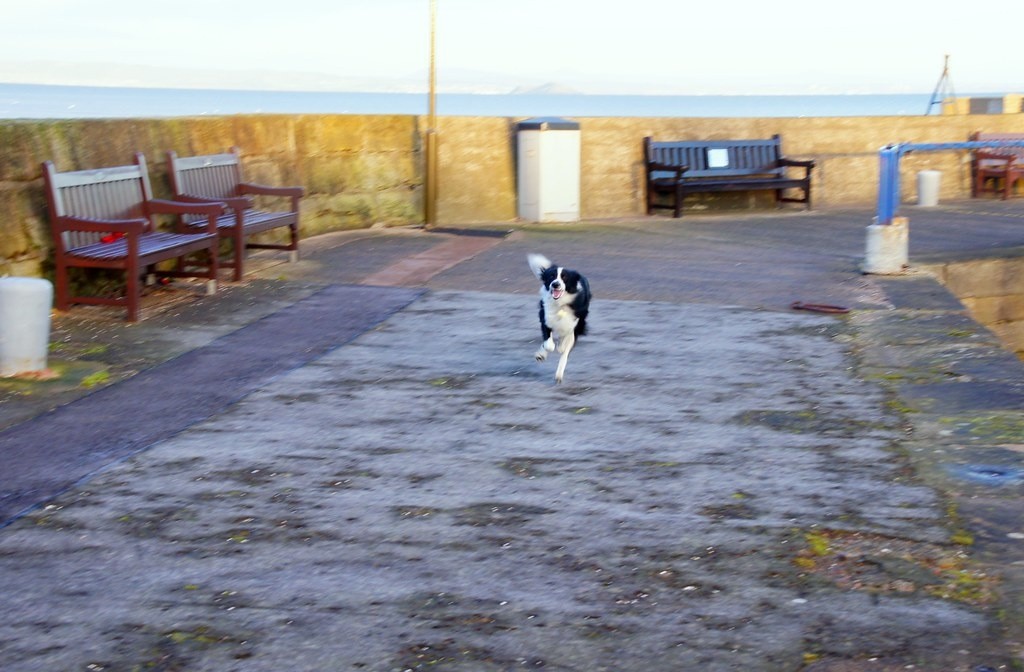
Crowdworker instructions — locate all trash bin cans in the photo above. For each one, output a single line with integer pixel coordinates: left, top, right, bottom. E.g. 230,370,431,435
516,116,581,222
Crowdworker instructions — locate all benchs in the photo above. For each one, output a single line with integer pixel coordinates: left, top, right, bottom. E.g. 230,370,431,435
975,131,1024,199
645,132,817,218
43,152,227,323
165,145,307,281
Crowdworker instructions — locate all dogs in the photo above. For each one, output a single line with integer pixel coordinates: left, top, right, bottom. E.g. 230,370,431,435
526,251,592,385
